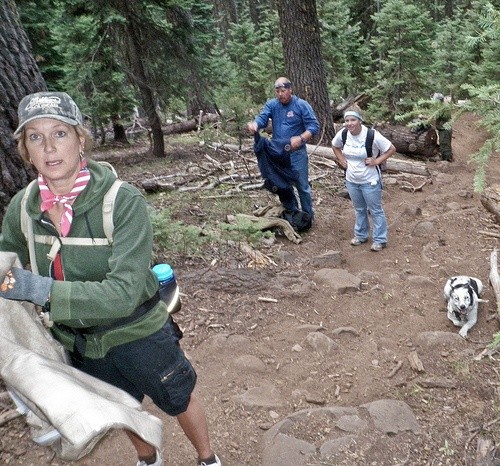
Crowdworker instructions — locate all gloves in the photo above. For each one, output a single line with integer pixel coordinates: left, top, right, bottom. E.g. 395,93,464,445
0,267,54,307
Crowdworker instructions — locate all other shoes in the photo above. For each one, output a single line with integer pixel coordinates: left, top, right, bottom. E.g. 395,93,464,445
137,446,164,466
197,455,221,466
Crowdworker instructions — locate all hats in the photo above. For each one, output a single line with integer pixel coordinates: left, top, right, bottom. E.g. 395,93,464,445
13,92,82,140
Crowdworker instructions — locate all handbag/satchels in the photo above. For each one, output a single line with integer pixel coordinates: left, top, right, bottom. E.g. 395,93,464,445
279,208,312,233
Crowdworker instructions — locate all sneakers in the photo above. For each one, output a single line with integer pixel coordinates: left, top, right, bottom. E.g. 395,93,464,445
350,237,368,245
370,243,386,251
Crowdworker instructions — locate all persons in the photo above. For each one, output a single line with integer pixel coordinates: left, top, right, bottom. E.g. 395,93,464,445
0,91,222,466
246,76,320,219
331,104,397,252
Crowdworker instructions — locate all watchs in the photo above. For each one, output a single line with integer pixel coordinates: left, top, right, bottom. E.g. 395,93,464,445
300,135,306,144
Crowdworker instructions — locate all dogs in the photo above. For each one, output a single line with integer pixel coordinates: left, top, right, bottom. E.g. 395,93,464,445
444,275,489,338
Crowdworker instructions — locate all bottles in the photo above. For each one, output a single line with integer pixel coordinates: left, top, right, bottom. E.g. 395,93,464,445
153,263,181,313
